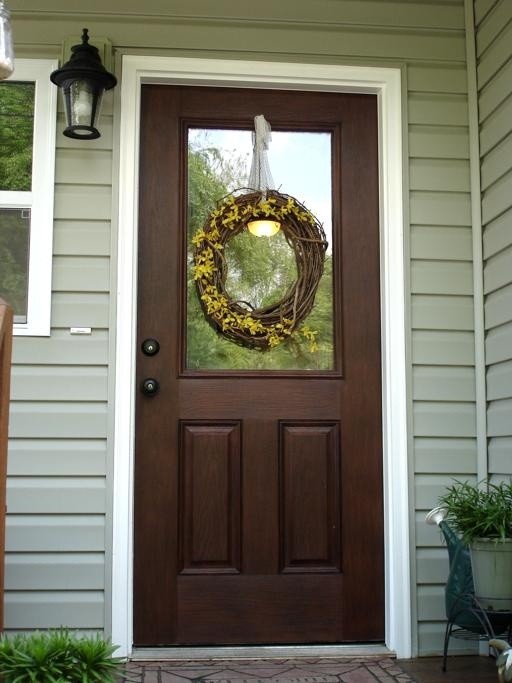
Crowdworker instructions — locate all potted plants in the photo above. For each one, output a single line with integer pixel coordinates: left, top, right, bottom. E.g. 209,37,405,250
438,474,511,614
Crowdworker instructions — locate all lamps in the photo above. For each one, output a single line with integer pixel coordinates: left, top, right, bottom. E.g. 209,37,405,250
46,20,121,141
244,216,282,239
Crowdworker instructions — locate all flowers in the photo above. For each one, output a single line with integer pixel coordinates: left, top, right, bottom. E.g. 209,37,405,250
191,188,328,353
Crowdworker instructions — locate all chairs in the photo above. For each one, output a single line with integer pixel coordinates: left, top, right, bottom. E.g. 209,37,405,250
435,518,512,676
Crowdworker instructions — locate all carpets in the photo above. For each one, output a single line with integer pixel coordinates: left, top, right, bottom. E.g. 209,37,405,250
119,653,419,681
